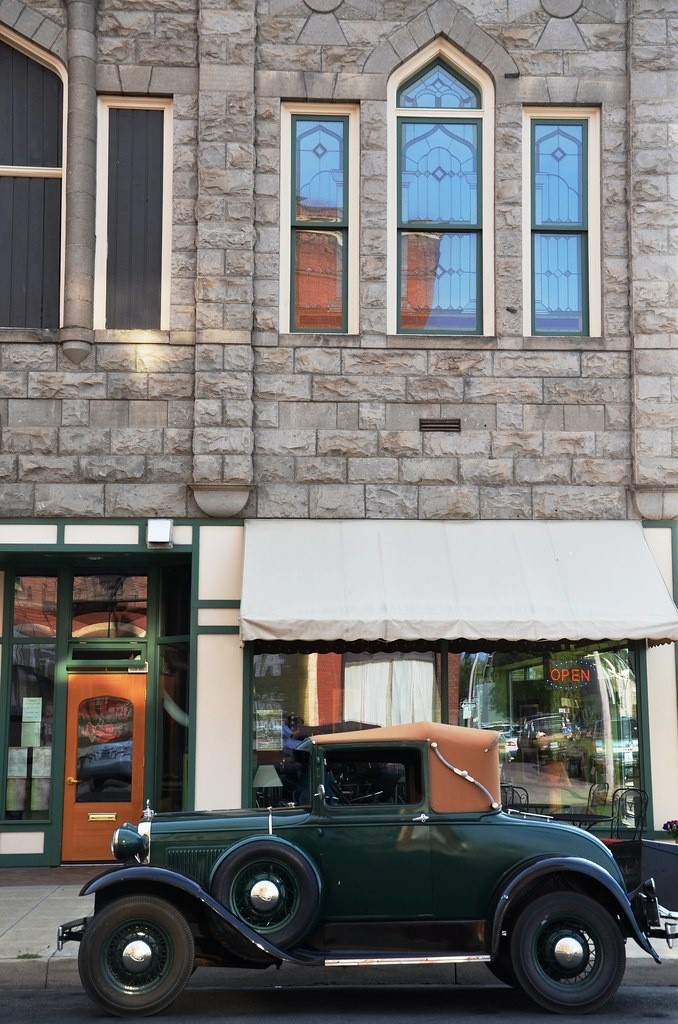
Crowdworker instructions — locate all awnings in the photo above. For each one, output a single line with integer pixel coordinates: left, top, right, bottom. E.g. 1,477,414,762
239,518,678,649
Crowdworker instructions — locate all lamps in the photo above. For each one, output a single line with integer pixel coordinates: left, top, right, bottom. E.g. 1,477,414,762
253,764,283,808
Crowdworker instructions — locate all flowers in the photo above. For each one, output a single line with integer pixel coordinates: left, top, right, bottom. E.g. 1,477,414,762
663,821,678,840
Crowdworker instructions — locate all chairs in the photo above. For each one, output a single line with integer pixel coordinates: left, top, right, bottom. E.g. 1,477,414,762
599,788,648,877
501,786,529,813
584,782,609,825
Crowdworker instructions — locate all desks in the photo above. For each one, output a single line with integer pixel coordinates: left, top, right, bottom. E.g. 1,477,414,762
539,814,613,831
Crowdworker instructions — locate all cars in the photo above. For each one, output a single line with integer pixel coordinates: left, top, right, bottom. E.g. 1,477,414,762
476,714,581,766
53,720,663,1017
